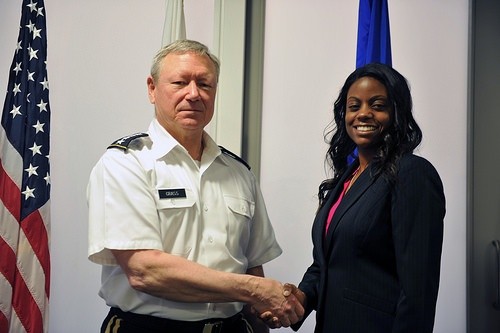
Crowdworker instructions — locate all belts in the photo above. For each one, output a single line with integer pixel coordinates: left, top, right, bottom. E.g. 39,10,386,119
110,303,250,333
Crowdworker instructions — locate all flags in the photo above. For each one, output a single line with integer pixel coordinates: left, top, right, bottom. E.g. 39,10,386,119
347,0,393,165
0,0,53,332
159,0,187,51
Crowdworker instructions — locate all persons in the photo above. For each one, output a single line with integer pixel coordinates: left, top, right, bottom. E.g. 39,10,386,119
85,38,306,333
251,63,447,333
